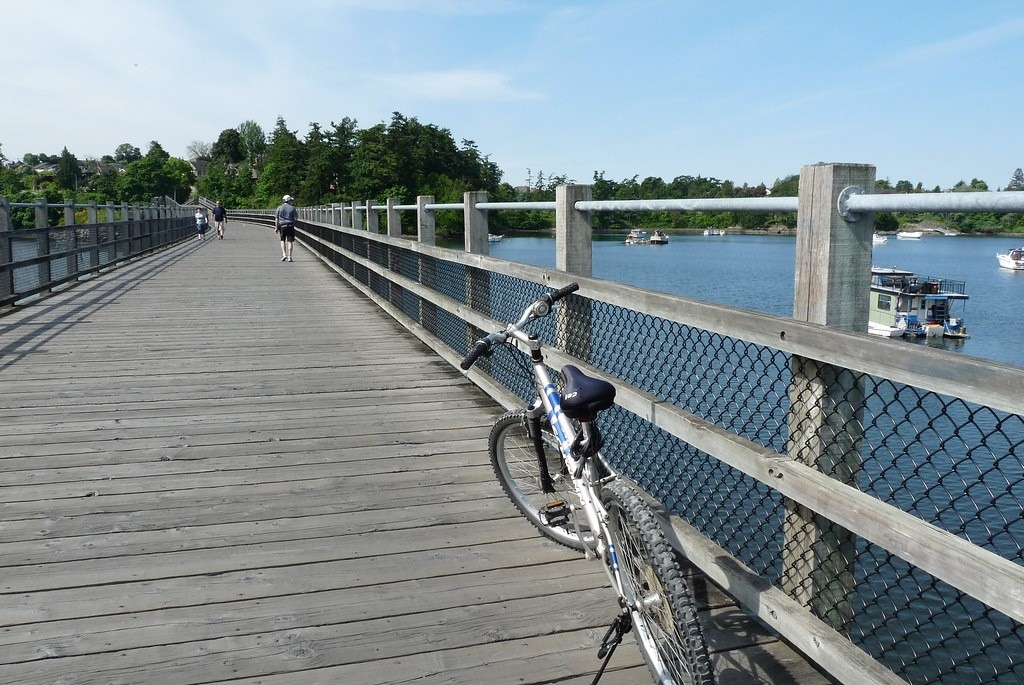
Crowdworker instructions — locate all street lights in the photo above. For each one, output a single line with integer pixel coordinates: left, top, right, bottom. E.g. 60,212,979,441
71,173,78,191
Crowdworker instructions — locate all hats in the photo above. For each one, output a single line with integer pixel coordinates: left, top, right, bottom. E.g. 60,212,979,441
283,194,294,202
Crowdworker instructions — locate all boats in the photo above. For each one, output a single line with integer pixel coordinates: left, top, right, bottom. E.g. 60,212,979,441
704,225,727,237
996,234,1024,270
867,264,973,340
896,225,924,239
935,227,961,237
488,233,505,242
625,227,648,244
649,227,669,244
873,229,889,244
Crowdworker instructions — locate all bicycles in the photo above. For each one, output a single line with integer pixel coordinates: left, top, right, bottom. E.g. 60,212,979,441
460,281,717,685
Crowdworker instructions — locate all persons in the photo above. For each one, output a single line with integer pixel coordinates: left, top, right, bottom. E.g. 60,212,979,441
195,208,208,240
211,201,227,239
275,195,298,262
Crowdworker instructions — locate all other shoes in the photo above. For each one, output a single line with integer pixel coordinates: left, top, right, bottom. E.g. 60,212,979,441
220,235,223,239
218,237,220,240
203,237,205,240
287,258,294,262
281,255,287,261
198,238,201,240
